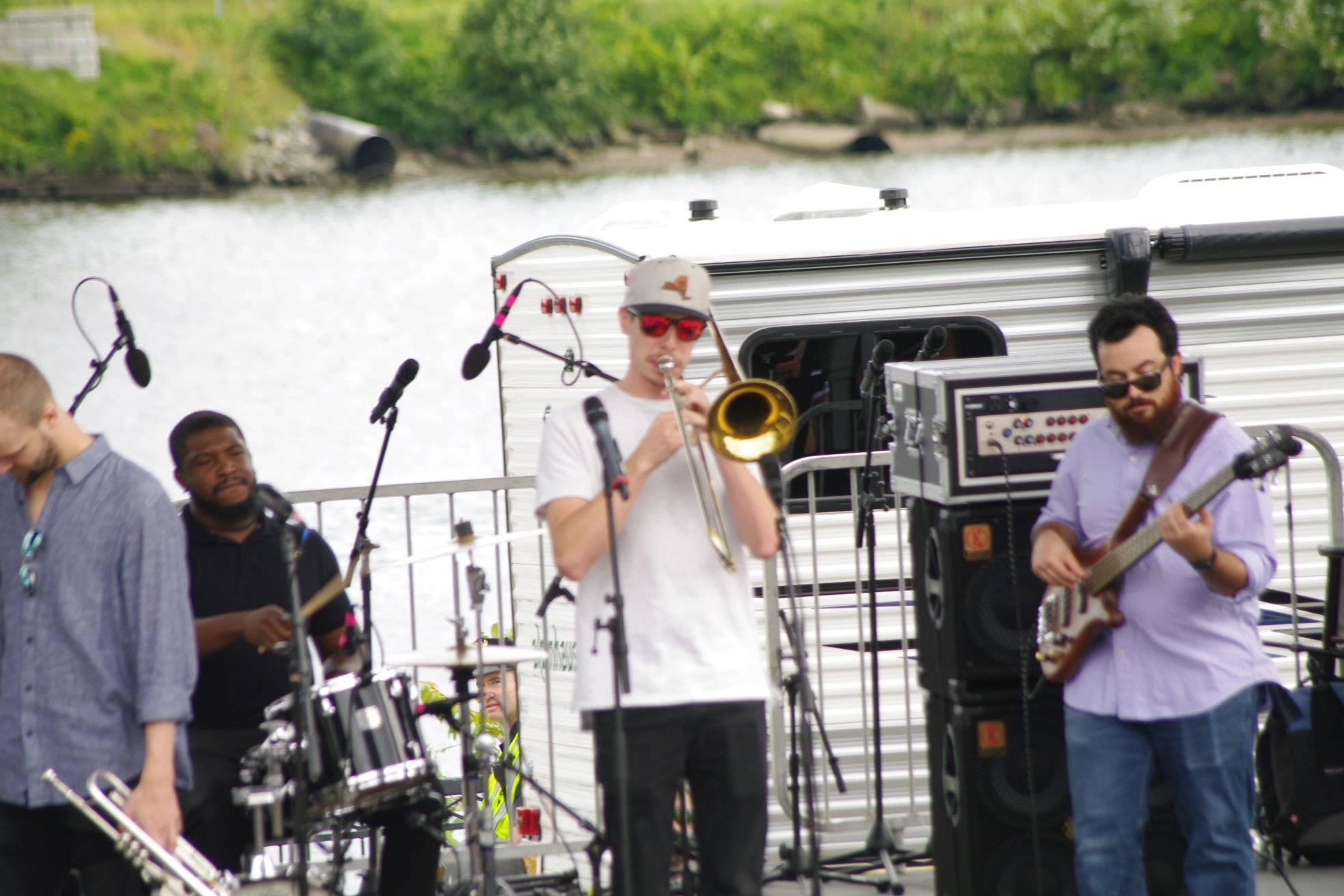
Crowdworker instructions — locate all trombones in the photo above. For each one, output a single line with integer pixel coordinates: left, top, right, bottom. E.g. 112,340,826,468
656,304,798,565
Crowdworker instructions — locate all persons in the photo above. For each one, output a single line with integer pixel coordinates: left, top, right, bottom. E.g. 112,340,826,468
1026,293,1278,896
0,354,202,896
471,636,523,842
537,256,783,896
169,410,443,896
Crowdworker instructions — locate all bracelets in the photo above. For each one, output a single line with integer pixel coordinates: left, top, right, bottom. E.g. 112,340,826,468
1192,545,1217,574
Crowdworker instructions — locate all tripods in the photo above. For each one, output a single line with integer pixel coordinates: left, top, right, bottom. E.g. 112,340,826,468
762,360,918,896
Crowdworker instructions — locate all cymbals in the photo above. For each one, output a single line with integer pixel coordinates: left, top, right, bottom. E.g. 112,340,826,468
368,528,547,569
388,644,551,668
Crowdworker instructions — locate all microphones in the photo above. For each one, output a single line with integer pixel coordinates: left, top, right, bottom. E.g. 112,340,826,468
462,283,523,380
254,484,303,525
370,359,419,423
109,285,151,388
536,573,563,616
415,692,478,716
338,607,357,657
758,453,783,515
584,397,630,499
860,341,894,398
916,325,947,361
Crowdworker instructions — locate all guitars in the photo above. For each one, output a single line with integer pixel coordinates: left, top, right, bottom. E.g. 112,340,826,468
1036,427,1301,683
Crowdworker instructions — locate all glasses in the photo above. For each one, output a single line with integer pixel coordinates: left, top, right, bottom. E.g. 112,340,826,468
19,529,43,594
628,307,707,342
1097,361,1171,399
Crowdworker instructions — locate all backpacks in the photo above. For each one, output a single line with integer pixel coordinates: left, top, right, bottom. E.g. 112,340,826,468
1255,674,1344,863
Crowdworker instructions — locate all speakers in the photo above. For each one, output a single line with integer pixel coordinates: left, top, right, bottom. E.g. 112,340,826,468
923,692,1191,896
906,497,1064,703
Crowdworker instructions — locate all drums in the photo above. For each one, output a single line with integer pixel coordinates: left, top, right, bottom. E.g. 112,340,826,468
259,667,435,818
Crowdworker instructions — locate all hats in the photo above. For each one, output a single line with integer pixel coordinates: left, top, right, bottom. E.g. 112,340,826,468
621,254,711,320
451,638,516,675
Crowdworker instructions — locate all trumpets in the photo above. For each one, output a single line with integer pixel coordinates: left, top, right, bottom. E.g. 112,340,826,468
38,767,242,896
